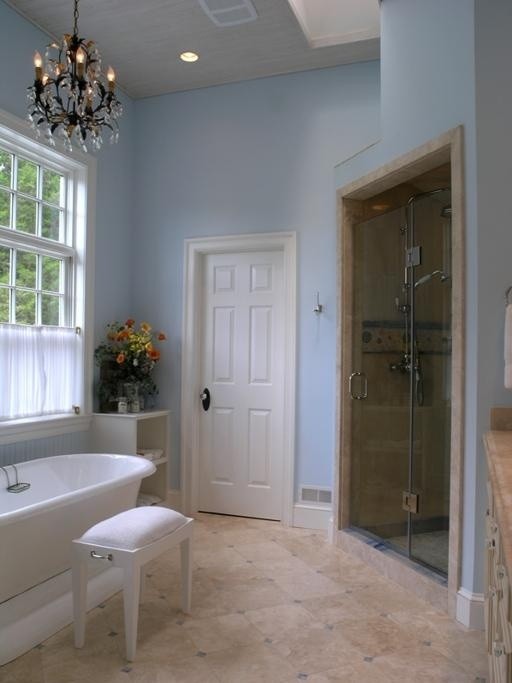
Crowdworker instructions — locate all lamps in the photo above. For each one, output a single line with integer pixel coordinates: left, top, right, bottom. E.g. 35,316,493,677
26,0,126,153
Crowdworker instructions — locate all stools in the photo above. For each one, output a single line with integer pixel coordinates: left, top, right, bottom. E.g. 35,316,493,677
72,504,196,663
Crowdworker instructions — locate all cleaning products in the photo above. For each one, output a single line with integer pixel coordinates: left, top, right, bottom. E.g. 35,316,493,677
117,396,129,413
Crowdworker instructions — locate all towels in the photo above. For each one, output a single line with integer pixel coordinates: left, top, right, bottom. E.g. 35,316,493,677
503,305,512,391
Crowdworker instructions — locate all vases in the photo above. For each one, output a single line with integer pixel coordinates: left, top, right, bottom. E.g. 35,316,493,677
116,369,144,413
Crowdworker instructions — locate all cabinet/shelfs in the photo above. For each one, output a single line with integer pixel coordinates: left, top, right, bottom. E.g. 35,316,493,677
482,407,512,683
93,409,170,507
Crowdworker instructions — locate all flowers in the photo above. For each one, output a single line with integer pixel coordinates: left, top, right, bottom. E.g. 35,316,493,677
93,319,168,413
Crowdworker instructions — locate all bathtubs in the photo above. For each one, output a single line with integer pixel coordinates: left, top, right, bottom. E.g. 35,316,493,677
1,453,158,667
